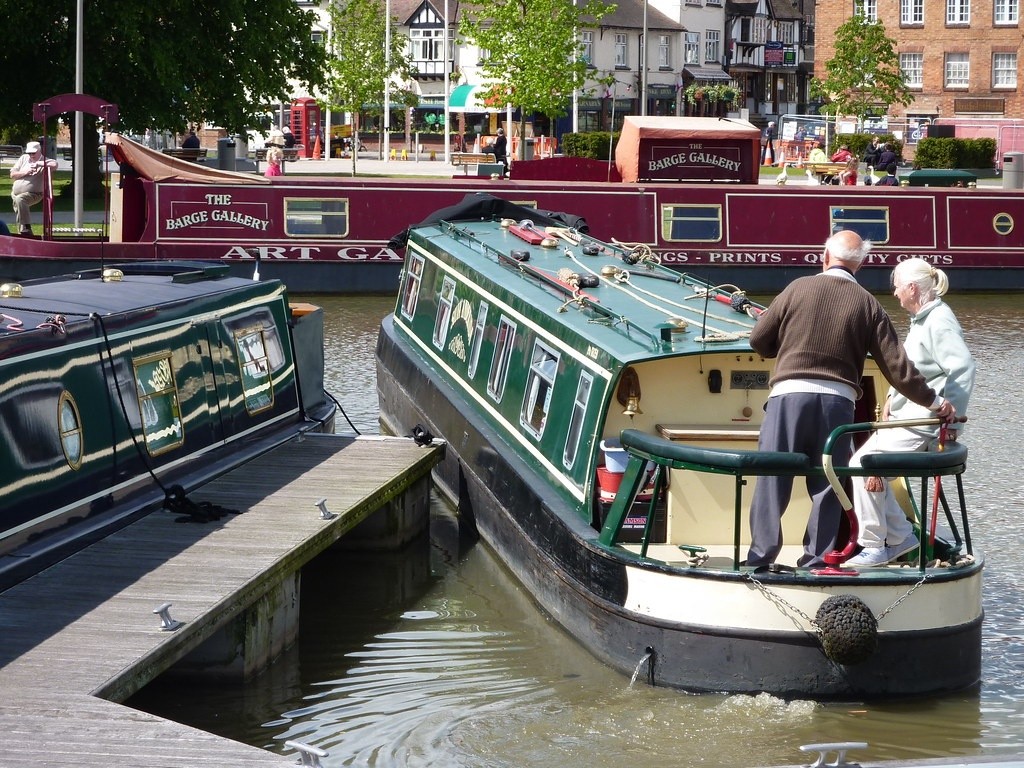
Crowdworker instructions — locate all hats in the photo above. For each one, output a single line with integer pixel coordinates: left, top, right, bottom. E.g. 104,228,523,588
496,128,504,135
26,142,41,153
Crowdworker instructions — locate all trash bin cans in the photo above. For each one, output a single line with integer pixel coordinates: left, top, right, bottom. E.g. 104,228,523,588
519,137,538,160
1003,152,1024,188
38,136,59,159
218,138,237,172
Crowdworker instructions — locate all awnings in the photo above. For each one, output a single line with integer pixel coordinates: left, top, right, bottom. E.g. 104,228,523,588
684,67,732,79
448,85,516,113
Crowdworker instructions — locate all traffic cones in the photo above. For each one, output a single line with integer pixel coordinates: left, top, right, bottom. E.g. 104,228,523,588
777,147,785,166
309,135,325,160
795,152,803,167
761,140,773,166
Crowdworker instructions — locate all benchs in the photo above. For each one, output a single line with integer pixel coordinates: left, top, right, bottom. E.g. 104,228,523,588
655,421,765,543
450,153,497,175
251,149,299,173
161,149,208,162
806,159,866,185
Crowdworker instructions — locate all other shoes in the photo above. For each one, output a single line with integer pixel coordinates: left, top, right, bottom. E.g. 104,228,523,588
797,555,828,568
821,181,829,185
20,224,32,235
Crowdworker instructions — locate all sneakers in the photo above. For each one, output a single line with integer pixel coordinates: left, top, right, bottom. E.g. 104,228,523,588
887,535,919,561
845,547,892,567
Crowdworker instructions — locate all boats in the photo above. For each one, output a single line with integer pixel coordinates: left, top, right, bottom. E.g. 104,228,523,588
0,263,336,593
374,194,985,701
0,91,1024,295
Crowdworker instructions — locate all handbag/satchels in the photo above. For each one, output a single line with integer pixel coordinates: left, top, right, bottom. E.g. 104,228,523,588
761,136,768,146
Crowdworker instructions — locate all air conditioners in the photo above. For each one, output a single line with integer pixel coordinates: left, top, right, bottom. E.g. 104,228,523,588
310,34,324,45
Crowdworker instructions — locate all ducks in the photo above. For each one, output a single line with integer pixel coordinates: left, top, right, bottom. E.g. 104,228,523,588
867,165,880,186
805,169,820,186
777,163,791,186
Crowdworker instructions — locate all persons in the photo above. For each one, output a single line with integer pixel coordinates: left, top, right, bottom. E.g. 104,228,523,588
874,144,896,171
809,141,834,185
482,138,494,152
766,122,775,135
267,125,285,148
283,127,296,148
831,144,852,163
11,142,58,235
839,258,975,566
839,158,860,185
748,231,957,570
876,163,898,186
265,147,284,176
863,137,883,168
182,132,199,161
493,128,507,173
864,175,872,186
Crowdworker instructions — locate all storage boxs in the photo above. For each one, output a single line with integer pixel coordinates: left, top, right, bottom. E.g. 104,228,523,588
599,437,656,472
597,498,666,544
